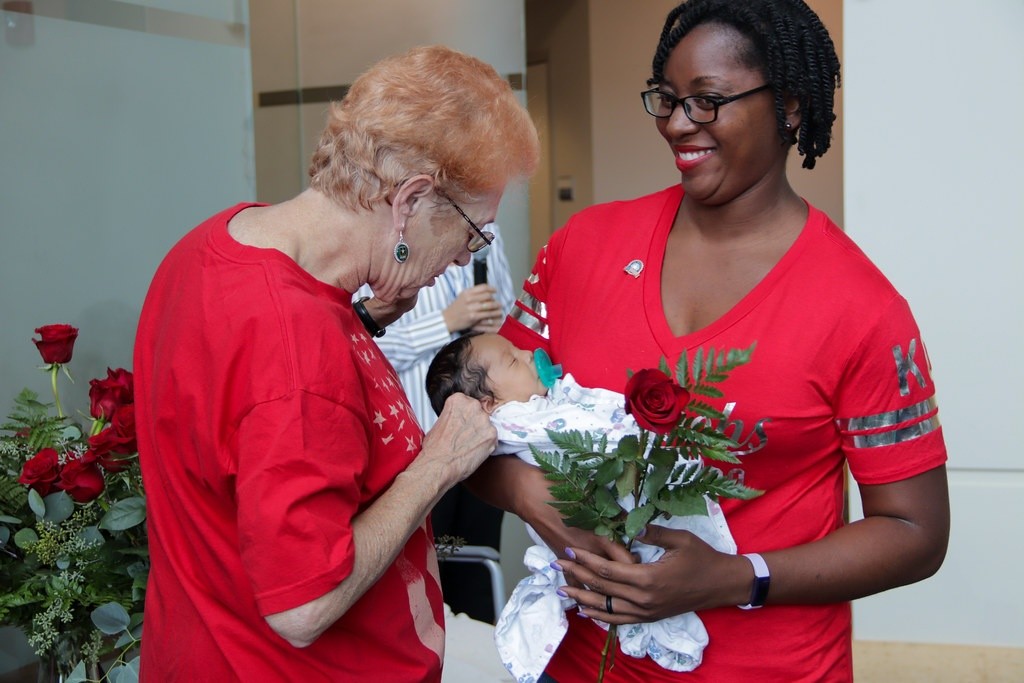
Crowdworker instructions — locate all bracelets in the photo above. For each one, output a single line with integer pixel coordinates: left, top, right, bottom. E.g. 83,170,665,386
351,296,385,338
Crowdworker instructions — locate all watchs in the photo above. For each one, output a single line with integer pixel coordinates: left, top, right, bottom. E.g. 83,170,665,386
738,551,770,609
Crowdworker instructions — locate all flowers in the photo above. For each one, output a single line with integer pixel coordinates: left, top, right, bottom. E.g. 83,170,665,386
527,367,718,683
0,323,151,683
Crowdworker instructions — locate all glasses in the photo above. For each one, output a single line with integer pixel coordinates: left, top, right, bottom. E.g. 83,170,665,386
400,179,495,252
640,83,772,124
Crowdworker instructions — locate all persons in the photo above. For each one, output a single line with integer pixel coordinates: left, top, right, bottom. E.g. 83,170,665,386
133,47,538,683
353,221,516,625
425,332,737,562
498,0,951,683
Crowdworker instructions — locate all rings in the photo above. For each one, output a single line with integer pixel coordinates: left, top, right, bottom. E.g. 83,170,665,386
606,597,612,613
